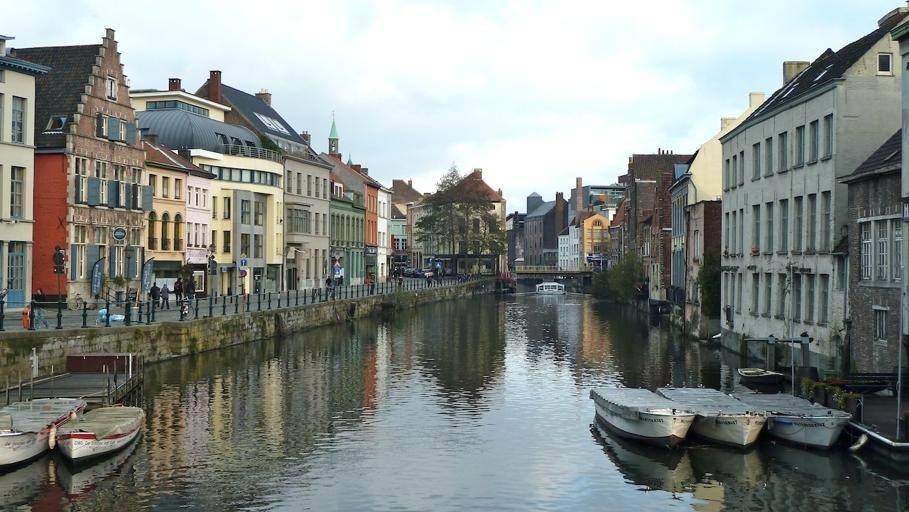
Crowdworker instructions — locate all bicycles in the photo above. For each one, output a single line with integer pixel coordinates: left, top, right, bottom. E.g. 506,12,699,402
179,297,195,320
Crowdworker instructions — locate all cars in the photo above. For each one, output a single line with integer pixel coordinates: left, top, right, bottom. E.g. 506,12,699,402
393,264,440,283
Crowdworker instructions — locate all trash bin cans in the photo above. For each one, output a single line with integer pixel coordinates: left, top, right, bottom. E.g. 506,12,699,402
130,306,139,322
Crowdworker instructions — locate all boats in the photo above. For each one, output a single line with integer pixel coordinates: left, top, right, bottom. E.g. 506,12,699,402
736,364,788,389
54,400,150,465
51,431,145,502
0,449,58,510
585,382,852,455
587,419,863,501
0,395,87,472
535,280,568,298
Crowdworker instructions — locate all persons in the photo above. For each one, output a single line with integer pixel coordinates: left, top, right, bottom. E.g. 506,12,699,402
147,282,161,312
325,275,332,295
391,268,400,280
174,277,184,306
427,273,433,288
34,305,50,330
160,284,172,309
338,275,343,286
186,275,196,306
